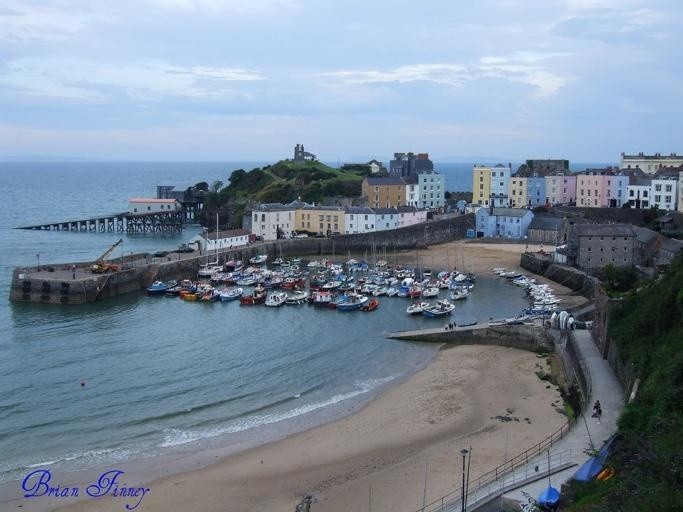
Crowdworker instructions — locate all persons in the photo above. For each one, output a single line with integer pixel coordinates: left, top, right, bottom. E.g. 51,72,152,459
595,399,601,409
597,404,602,423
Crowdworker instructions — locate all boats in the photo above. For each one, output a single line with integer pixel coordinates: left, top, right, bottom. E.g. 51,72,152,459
488,262,562,316
